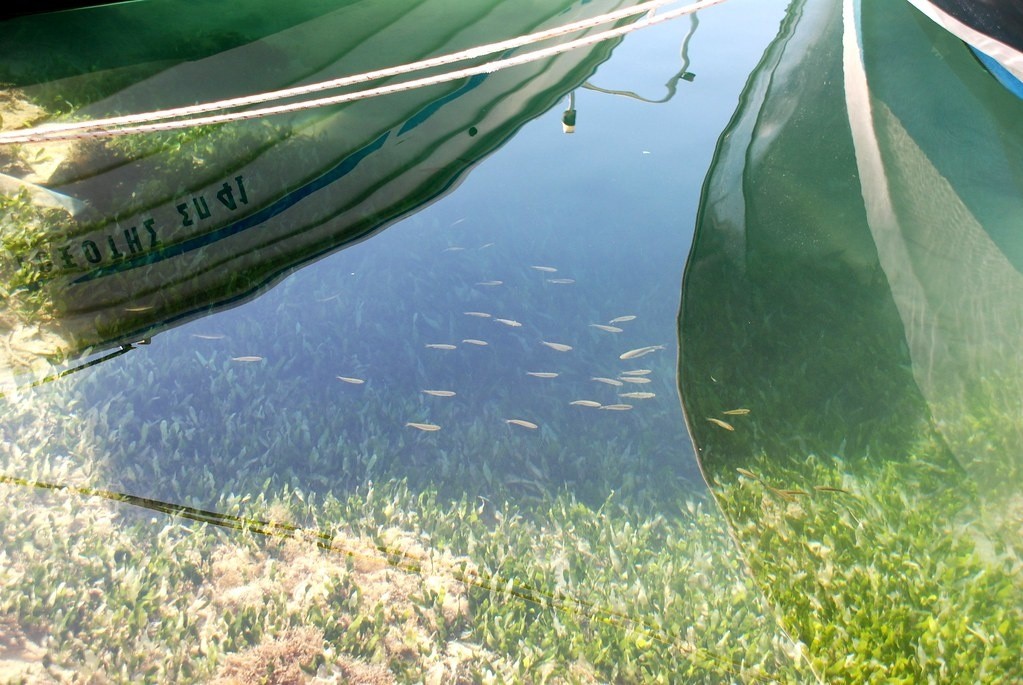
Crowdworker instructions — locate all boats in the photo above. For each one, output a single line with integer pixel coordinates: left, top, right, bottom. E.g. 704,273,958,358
677,0,1023,685
0,1,747,393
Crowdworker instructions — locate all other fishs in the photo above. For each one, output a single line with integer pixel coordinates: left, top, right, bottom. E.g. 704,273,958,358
121,216,868,503
